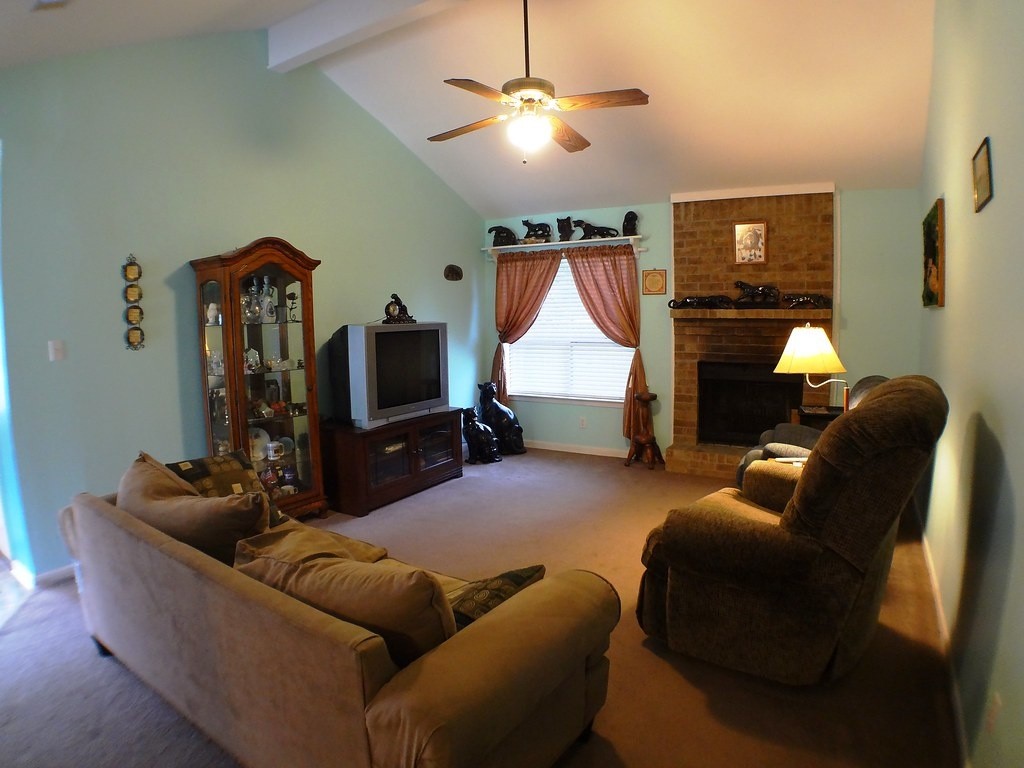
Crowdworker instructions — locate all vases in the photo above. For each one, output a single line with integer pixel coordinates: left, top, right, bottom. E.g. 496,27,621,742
922,199,944,307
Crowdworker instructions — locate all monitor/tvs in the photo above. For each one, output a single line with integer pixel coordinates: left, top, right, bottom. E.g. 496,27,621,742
327,321,449,431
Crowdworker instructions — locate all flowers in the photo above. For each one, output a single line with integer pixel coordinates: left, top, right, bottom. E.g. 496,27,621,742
286,293,299,321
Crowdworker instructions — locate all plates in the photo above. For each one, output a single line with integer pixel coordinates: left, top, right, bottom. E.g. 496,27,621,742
248,428,271,461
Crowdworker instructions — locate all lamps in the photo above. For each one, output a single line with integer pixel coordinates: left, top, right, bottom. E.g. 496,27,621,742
773,321,850,413
506,105,550,154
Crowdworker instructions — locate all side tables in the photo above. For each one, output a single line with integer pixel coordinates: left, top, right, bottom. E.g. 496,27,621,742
799,405,844,431
766,458,807,468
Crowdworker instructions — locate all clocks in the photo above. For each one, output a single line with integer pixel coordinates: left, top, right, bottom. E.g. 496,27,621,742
382,293,416,324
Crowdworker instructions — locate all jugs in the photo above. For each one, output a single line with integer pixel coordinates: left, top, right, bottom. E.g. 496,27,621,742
240,293,264,324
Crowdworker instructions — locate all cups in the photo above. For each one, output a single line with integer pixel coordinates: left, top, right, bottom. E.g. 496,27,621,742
282,485,298,496
267,441,286,460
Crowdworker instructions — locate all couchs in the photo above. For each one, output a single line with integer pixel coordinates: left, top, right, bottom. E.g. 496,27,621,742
635,374,949,690
57,493,621,768
736,375,891,491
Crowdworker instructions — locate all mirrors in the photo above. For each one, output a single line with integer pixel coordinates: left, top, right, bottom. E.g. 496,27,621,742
972,137,993,213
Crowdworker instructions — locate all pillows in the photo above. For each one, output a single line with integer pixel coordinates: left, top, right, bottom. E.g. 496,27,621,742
165,448,290,529
446,565,547,632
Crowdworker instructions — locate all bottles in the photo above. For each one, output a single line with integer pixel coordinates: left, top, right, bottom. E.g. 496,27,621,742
262,461,279,489
253,275,277,323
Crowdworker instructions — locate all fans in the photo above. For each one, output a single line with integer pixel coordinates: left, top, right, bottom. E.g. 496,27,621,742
426,0,650,152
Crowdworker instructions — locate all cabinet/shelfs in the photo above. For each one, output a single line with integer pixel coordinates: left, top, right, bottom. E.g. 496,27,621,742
318,406,464,517
188,238,328,518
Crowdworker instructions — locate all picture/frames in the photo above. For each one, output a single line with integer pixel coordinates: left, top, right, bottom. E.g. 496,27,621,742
732,221,769,265
